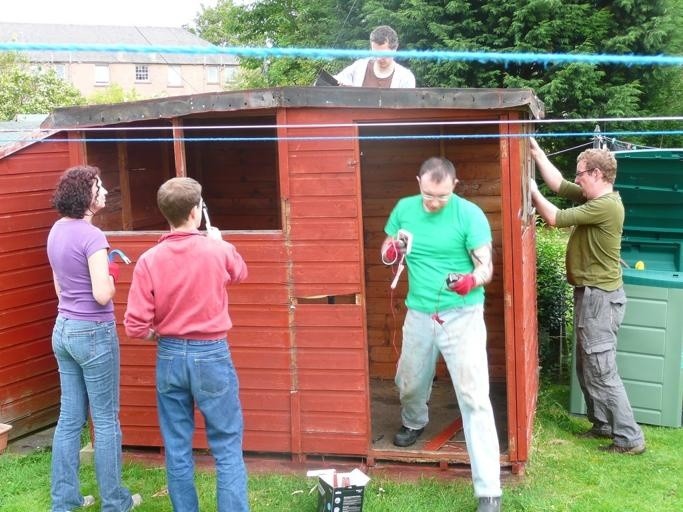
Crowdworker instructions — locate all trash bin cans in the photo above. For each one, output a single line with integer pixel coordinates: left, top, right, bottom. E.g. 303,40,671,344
570,148,683,427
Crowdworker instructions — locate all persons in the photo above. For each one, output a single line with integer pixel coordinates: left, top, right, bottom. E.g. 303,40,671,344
122,176,249,512
45,165,143,511
312,25,416,89
381,157,504,512
527,134,647,456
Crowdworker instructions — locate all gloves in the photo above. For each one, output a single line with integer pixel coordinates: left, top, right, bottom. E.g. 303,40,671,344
446,274,476,295
386,240,407,262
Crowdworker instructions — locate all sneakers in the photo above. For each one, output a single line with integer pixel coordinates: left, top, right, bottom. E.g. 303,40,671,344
477,496,500,512
130,494,141,509
572,429,616,440
395,426,423,447
84,494,94,508
596,444,646,456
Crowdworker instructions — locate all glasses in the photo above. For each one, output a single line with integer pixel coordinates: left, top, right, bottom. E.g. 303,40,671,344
419,181,449,202
